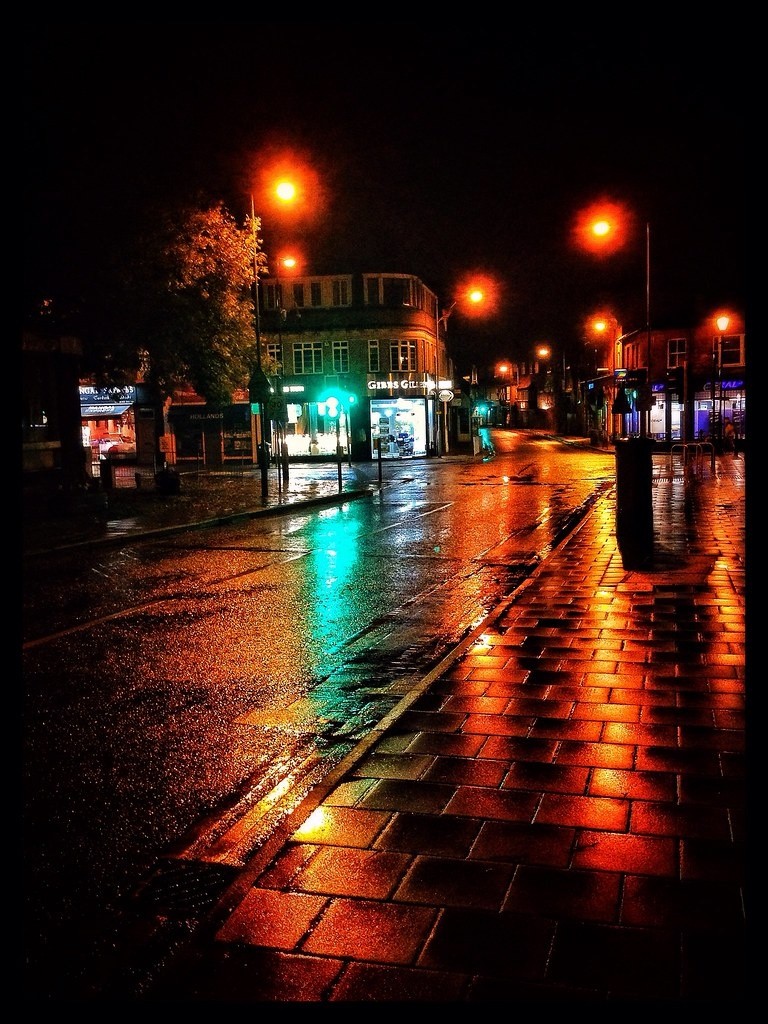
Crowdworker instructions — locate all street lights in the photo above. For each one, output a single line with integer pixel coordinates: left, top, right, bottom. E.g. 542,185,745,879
715,315,730,455
582,200,654,436
592,319,617,444
435,286,481,458
250,177,298,499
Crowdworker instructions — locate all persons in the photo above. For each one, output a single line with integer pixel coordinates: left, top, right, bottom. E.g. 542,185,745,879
722,417,736,450
601,425,609,450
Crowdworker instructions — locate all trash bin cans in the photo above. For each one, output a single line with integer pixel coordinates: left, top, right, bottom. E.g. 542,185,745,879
611,435,656,513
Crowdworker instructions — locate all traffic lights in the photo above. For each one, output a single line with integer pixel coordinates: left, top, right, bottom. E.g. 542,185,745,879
325,394,340,419
